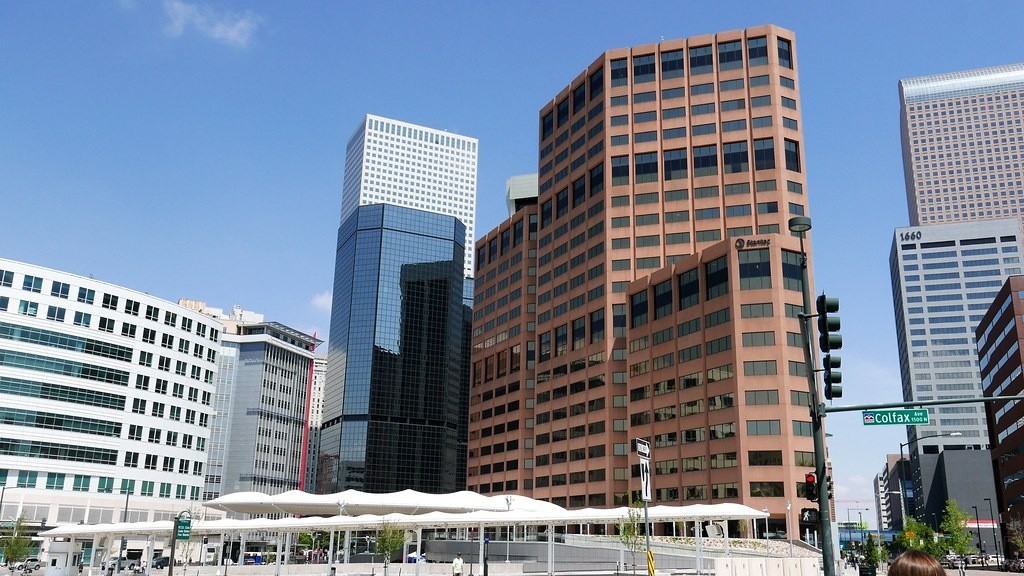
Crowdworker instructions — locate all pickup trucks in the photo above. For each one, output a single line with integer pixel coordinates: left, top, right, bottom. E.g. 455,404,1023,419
102,556,139,571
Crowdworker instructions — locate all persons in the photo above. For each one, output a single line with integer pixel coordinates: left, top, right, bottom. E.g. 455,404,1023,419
134,560,147,574
78,561,83,576
189,557,192,567
887,549,947,576
452,552,463,576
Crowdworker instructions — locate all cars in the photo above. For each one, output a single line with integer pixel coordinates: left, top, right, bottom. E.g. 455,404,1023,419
941,555,1004,570
152,557,176,569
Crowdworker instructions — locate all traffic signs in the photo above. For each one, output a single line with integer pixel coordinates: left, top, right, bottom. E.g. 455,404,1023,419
636,442,650,457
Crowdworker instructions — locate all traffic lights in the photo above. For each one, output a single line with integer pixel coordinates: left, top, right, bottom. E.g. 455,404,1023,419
805,474,816,501
826,476,831,499
823,354,843,400
816,290,843,353
802,508,819,527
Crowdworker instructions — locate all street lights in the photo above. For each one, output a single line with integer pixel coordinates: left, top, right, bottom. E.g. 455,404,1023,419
847,508,870,567
984,498,1000,570
931,512,939,551
971,505,985,571
900,433,962,519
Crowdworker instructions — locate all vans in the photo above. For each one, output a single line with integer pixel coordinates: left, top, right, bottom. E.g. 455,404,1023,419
11,558,41,570
